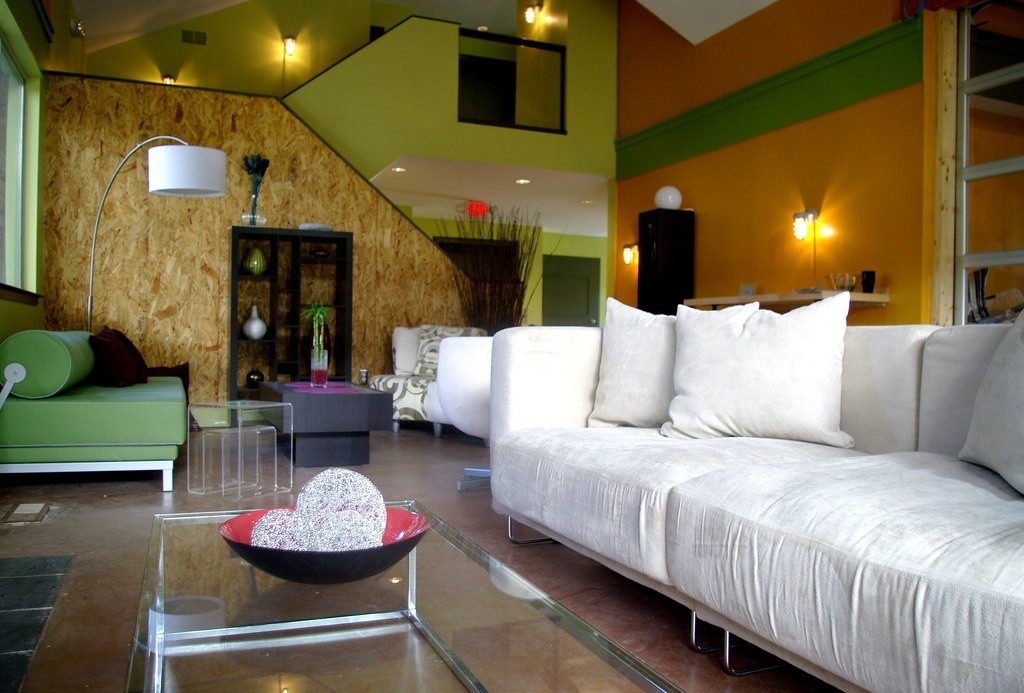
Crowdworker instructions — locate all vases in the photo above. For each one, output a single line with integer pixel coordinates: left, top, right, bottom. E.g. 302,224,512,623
241,191,267,227
242,247,268,277
310,349,329,388
246,367,265,389
242,305,268,340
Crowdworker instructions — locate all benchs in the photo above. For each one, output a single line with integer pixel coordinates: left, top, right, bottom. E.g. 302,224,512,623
0,330,187,492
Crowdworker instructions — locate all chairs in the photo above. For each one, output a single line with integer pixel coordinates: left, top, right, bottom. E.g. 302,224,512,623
967,266,1024,323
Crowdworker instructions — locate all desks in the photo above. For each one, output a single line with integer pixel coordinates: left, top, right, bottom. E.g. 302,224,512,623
684,290,891,311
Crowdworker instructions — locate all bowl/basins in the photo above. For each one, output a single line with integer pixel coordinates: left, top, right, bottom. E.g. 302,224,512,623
830,272,860,292
218,507,431,584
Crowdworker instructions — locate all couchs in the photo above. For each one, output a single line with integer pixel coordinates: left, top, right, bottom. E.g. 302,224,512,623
367,325,455,438
490,323,1024,693
436,334,492,448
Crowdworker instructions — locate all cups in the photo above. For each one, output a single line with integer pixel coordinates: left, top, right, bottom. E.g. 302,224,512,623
862,271,875,293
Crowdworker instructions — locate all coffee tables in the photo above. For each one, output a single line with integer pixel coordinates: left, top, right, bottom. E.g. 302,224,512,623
258,381,393,468
124,500,684,693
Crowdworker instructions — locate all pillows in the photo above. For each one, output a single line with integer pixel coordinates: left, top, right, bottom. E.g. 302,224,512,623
147,362,190,407
957,308,1024,495
660,289,855,450
89,325,138,387
586,296,760,429
113,329,149,382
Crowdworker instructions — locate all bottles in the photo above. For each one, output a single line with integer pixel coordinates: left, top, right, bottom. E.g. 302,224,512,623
243,306,267,340
244,248,267,275
246,369,264,388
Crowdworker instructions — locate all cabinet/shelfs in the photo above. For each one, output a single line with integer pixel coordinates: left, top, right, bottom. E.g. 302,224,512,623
638,207,696,316
215,225,354,429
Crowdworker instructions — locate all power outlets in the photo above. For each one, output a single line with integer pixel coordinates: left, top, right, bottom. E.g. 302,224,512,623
358,368,368,385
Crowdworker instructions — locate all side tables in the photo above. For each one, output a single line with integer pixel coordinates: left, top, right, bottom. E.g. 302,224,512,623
186,398,295,501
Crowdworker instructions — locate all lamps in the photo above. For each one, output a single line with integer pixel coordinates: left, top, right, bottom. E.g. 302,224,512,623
623,242,638,265
161,73,175,84
455,199,497,217
792,208,818,241
524,0,544,24
86,134,227,331
282,34,297,56
